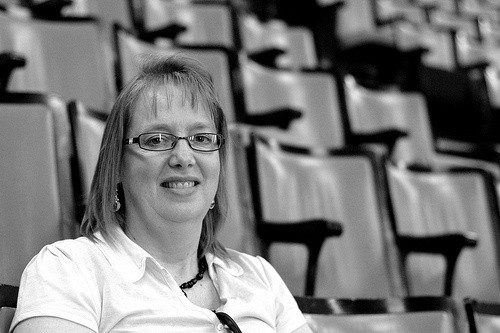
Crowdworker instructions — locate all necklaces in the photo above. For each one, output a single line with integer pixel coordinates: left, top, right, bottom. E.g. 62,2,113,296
177,261,212,293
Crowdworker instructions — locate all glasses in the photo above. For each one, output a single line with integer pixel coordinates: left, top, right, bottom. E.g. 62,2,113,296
122,132,226,152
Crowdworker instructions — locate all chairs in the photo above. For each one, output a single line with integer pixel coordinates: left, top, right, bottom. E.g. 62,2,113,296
0,0,500,333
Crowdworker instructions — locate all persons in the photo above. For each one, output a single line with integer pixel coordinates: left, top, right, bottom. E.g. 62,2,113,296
6,0,93,23
9,55,314,333
